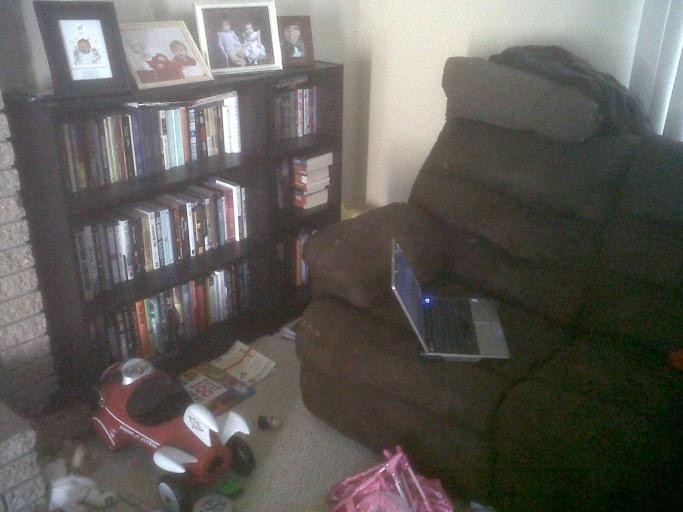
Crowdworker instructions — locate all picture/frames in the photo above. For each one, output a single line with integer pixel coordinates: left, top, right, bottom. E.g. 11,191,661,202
28,0,313,101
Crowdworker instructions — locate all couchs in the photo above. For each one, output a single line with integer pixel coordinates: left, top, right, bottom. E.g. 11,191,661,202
296,120,682,512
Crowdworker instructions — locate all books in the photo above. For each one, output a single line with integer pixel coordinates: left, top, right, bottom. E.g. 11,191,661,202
92,258,251,366
272,76,332,286
60,88,239,194
72,178,248,299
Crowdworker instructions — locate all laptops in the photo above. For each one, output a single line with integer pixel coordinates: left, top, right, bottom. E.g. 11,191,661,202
391,237,511,363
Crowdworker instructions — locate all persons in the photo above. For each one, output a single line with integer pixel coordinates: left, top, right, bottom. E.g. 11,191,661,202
241,24,267,65
168,39,197,66
282,24,304,58
72,38,101,67
215,18,248,66
129,39,169,73
